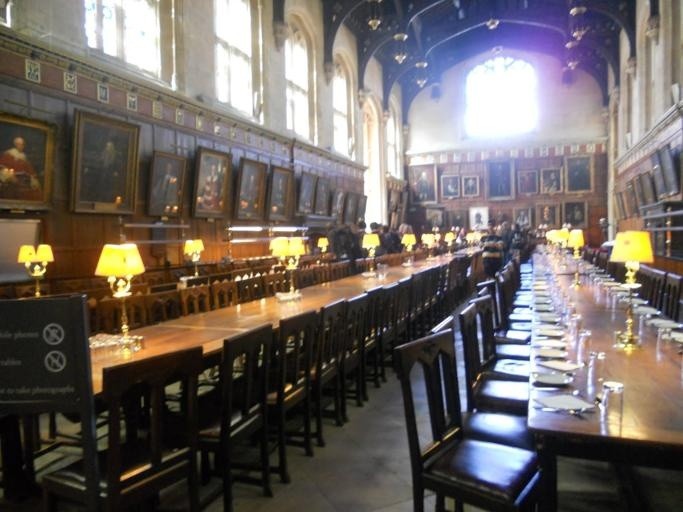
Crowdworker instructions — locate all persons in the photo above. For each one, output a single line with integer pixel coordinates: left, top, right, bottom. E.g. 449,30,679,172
202,163,224,209
415,171,430,200
325,214,526,273
545,172,559,194
152,163,180,215
0,136,42,202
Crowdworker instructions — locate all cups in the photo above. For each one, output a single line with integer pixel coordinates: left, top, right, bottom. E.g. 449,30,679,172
657,328,672,362
600,381,624,426
377,264,388,282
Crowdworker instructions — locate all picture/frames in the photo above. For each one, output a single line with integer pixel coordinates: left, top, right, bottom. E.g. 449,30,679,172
408,154,596,232
194,145,233,220
69,107,141,217
0,110,58,214
316,174,367,227
1,242,683,511
268,165,293,221
148,150,187,218
298,170,318,215
235,157,269,221
614,144,681,221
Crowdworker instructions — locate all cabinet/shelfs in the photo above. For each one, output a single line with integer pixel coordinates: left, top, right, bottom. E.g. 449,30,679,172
638,200,683,262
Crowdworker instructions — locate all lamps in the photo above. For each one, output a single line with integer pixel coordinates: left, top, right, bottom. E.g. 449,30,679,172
273,227,298,232
561,0,593,71
229,226,265,232
367,0,429,88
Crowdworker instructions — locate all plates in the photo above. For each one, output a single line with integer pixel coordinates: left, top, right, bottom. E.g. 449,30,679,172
532,279,594,411
605,281,683,345
